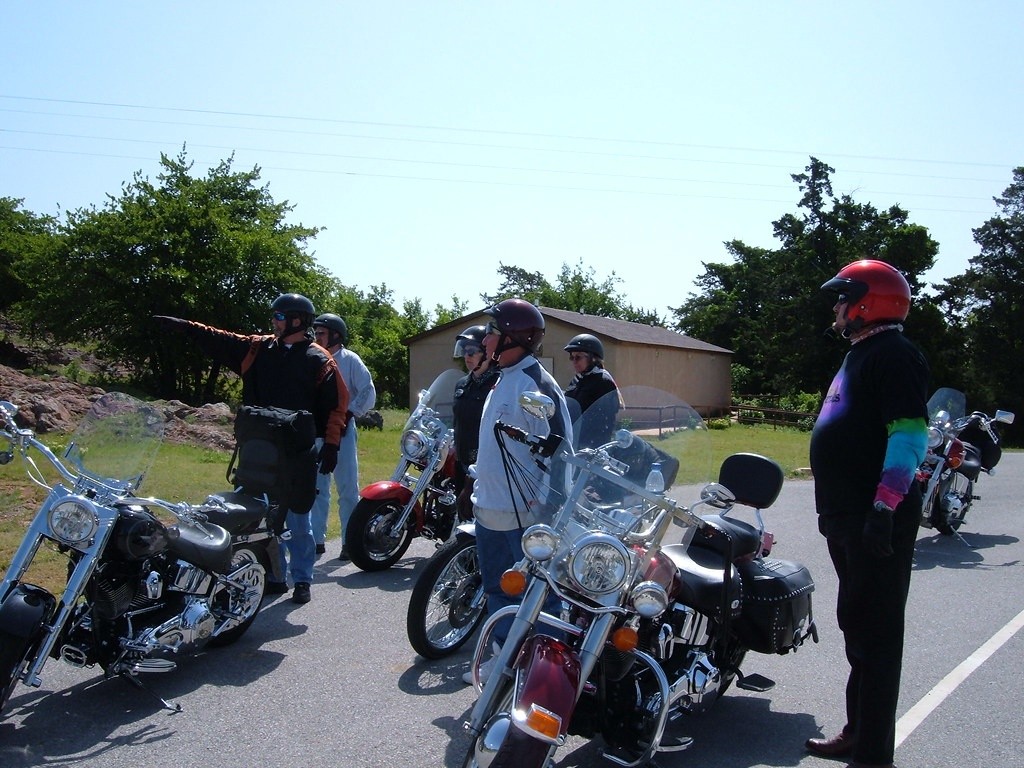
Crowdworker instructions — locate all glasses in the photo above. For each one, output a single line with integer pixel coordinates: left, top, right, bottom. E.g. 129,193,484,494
570,354,589,360
460,349,482,357
274,313,286,321
315,332,329,336
484,321,502,336
839,294,849,305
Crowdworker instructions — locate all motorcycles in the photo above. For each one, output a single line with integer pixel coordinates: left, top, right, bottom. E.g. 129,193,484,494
343,367,684,660
913,386,1015,549
0,390,293,725
461,383,820,768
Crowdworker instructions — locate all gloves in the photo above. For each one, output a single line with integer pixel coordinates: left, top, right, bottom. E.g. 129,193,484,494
818,513,829,538
153,316,186,333
318,443,339,476
862,505,894,557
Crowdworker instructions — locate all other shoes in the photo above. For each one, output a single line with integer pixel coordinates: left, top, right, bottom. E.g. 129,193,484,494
340,546,351,561
461,641,500,686
316,544,325,554
293,582,311,604
263,582,289,596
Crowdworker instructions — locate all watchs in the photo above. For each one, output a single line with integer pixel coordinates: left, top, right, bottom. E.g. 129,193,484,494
873,499,892,513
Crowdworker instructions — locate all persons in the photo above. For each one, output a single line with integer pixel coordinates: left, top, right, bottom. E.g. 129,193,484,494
311,310,376,563
555,328,625,500
805,257,930,768
448,318,499,525
463,298,566,683
152,294,350,604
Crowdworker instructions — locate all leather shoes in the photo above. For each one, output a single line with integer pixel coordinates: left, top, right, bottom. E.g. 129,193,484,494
806,732,853,757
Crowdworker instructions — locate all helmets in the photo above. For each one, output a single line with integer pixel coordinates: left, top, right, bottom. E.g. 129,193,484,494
483,299,545,351
456,325,487,349
564,334,604,360
273,294,315,314
314,313,347,338
820,259,911,327
